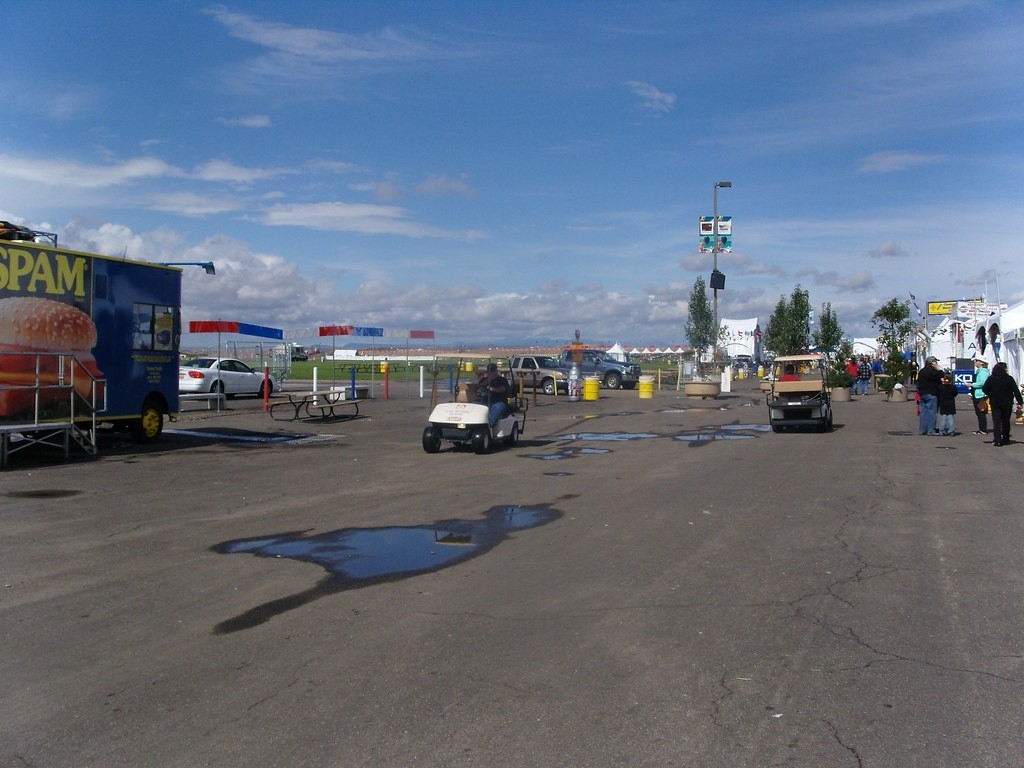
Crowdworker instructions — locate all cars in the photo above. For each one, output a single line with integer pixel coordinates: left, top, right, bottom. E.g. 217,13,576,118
178,357,276,399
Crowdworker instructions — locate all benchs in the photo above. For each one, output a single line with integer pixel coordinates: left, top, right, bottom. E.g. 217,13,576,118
335,366,407,372
759,380,827,409
177,393,226,414
309,400,364,423
269,399,320,421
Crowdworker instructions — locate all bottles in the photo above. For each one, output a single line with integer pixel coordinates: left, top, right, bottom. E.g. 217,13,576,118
568,362,580,401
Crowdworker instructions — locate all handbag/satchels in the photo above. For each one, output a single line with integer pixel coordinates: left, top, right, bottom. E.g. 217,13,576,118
1016,403,1023,418
868,379,870,383
978,399,988,414
857,377,860,383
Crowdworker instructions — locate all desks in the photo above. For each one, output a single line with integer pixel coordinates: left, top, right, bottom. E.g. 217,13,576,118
362,364,379,373
388,363,400,372
337,363,361,372
279,390,349,423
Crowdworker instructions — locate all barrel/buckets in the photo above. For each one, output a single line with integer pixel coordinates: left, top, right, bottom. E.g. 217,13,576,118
458,361,464,371
738,368,743,379
466,362,473,371
639,376,654,398
380,361,388,372
583,378,599,400
758,366,765,377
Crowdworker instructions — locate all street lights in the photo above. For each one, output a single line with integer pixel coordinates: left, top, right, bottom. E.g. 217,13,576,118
712,181,733,372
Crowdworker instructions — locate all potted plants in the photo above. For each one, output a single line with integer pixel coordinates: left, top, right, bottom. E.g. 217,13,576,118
683,275,736,400
876,352,911,403
824,341,855,402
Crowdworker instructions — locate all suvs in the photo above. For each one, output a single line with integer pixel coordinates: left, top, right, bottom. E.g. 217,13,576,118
557,349,642,390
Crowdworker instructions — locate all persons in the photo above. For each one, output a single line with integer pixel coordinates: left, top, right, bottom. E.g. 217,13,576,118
909,358,919,386
988,323,1001,362
872,357,884,389
857,357,872,395
779,363,801,382
848,355,859,395
966,355,990,435
479,363,510,389
902,359,909,387
982,362,1023,446
916,356,942,436
938,372,958,437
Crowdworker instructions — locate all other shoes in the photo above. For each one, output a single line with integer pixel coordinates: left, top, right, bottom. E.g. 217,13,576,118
1002,440,1011,444
918,432,926,435
927,432,939,435
993,440,1002,446
949,432,954,435
939,433,946,435
973,431,987,435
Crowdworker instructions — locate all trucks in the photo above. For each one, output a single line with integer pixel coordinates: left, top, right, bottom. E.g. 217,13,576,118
0,238,184,440
273,342,309,362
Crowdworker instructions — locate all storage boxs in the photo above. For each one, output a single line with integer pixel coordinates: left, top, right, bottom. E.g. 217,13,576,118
457,383,479,403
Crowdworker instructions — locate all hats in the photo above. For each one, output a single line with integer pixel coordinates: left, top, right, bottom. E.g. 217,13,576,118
487,364,497,372
926,356,940,364
971,355,989,363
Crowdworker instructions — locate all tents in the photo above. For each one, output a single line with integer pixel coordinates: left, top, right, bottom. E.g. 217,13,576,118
629,347,684,361
605,342,630,363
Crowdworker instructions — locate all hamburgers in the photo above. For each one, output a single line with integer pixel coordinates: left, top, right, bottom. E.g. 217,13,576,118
0,296,104,418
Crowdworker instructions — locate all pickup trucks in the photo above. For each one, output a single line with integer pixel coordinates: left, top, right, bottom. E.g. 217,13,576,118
474,354,568,395
